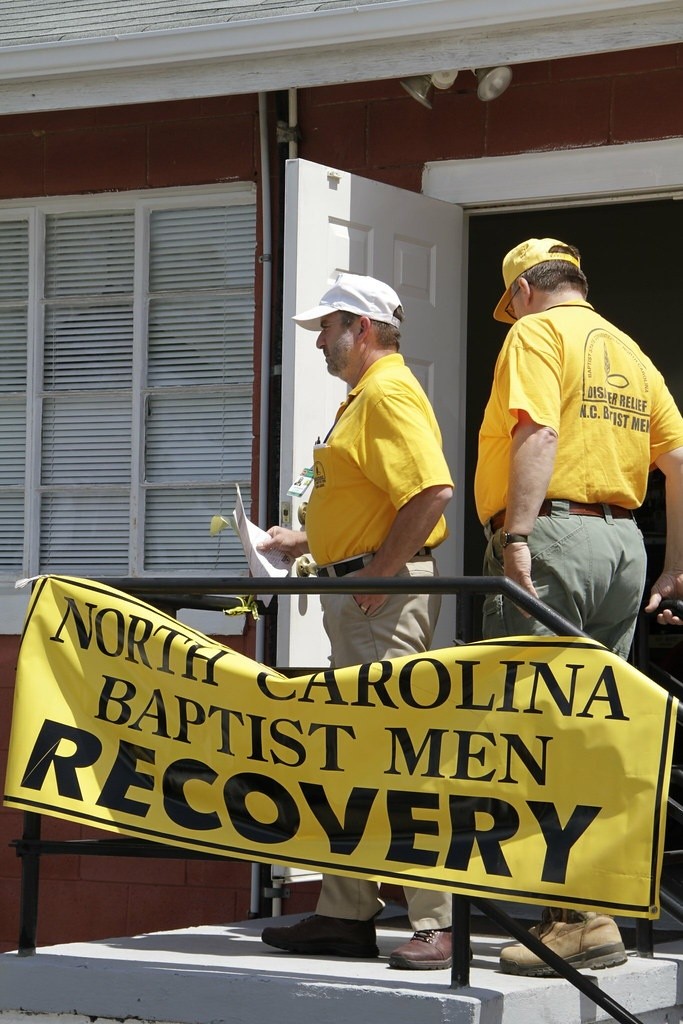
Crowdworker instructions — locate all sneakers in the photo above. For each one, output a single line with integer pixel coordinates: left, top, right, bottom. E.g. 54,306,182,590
499,912,627,976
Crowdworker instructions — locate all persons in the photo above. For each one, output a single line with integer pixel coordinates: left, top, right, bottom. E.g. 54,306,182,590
257,275,457,972
294,477,303,485
473,238,683,977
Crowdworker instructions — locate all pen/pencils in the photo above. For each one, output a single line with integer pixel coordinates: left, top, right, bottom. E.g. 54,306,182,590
314,437,321,446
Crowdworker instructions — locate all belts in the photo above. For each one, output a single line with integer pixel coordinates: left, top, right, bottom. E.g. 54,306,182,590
491,499,633,534
317,547,431,577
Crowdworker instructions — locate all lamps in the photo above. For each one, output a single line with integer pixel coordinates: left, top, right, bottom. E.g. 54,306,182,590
399,66,513,110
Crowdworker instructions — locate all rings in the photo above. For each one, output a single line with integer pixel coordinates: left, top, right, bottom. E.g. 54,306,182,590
360,605,366,610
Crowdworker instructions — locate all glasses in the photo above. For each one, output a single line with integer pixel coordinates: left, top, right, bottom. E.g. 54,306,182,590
505,282,536,322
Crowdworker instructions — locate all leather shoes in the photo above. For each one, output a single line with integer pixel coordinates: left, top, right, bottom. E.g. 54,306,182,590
388,925,473,969
261,915,379,957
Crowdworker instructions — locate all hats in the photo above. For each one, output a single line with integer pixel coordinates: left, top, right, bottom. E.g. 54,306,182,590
291,274,404,331
493,238,580,323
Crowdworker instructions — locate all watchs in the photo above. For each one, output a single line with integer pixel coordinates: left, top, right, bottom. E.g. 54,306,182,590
500,530,529,548
